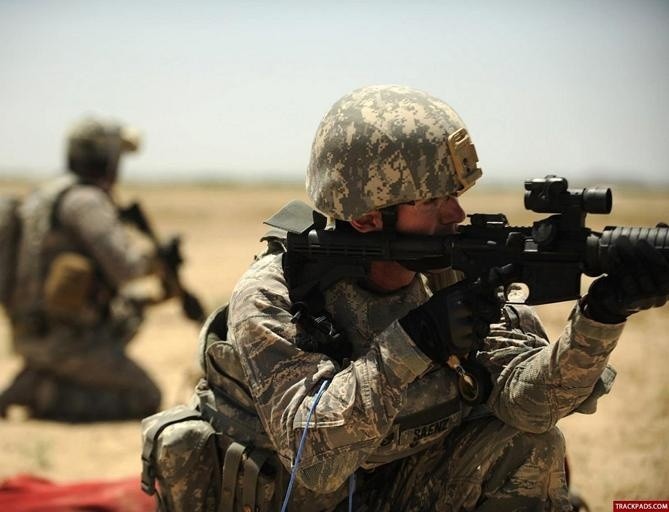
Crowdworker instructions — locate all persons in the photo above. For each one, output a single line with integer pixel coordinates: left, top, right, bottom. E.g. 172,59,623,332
137,81,668,512
0,112,180,424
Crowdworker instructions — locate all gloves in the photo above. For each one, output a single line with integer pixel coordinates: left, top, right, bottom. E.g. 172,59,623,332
158,238,183,272
396,279,504,379
584,222,669,324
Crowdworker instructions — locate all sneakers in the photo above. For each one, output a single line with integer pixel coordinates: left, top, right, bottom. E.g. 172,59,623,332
0,364,50,420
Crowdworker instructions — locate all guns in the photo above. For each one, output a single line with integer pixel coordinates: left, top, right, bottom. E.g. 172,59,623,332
116,200,206,330
282,173,669,323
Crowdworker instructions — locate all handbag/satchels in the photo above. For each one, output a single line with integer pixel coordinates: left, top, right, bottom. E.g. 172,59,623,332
140,405,223,512
42,250,97,312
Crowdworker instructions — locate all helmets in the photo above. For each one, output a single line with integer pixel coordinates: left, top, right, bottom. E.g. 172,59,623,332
67,119,138,173
304,82,482,223
